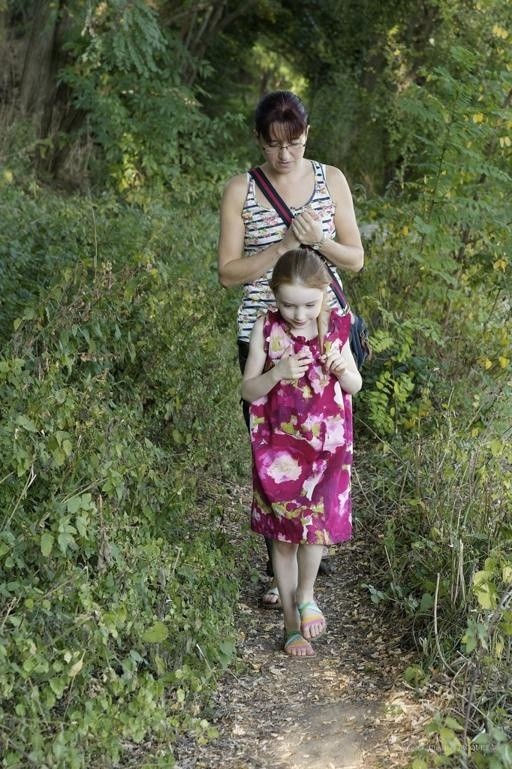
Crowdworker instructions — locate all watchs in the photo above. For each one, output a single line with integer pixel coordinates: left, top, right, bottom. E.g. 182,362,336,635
311,233,327,252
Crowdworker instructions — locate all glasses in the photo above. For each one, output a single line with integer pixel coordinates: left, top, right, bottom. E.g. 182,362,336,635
256,126,307,153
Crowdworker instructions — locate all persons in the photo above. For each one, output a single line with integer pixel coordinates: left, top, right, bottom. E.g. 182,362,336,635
238,248,363,660
217,87,365,611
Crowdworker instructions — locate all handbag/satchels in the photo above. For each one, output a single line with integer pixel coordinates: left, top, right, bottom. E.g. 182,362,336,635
348,313,371,370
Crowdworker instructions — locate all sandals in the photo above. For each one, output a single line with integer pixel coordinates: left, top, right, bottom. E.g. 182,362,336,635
284,631,316,658
299,601,326,641
263,586,281,608
